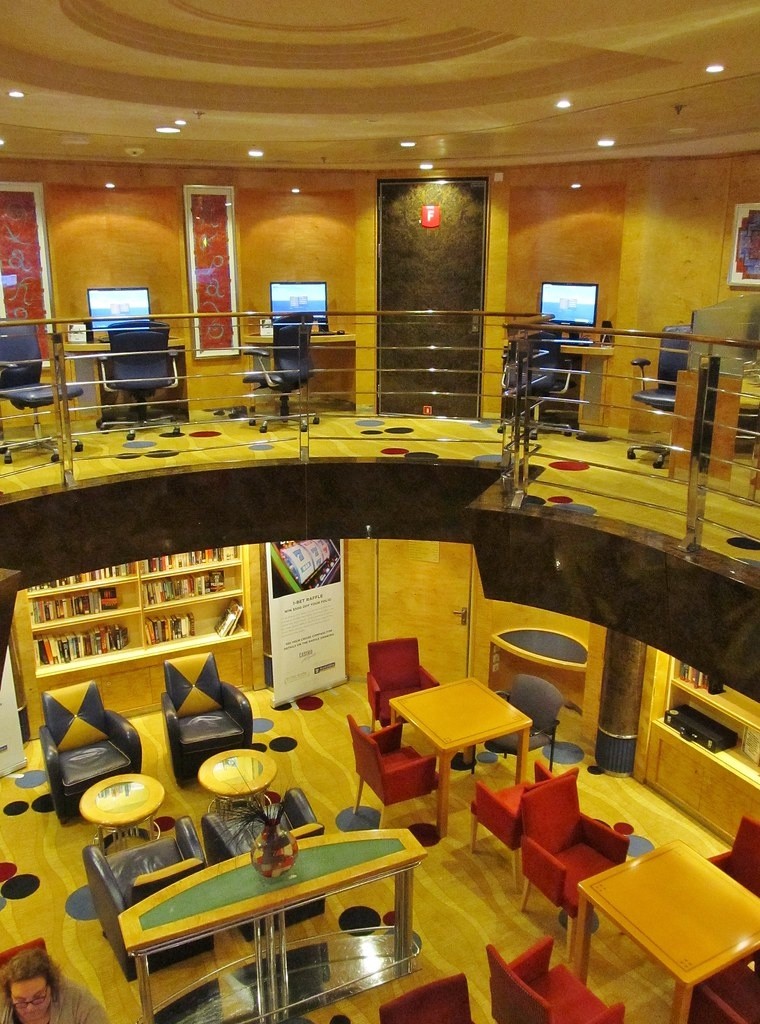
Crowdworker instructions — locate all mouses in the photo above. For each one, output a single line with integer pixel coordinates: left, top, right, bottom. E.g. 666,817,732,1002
337,330,345,335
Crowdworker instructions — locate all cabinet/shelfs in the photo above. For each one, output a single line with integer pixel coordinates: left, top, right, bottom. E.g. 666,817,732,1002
13,545,259,742
643,638,760,852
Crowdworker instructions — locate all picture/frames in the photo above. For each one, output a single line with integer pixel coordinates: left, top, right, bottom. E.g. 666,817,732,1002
0,182,57,371
183,185,241,359
727,203,760,287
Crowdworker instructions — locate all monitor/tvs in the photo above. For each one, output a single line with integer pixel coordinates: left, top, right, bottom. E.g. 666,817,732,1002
541,282,598,337
271,282,327,325
87,287,152,330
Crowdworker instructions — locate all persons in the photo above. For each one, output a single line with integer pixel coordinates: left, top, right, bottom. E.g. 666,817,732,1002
0,950,107,1024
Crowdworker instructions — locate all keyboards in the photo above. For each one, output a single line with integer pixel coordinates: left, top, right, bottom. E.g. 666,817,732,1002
98,337,111,343
310,332,335,336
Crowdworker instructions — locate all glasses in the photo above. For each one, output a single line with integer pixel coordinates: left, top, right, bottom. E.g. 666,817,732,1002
10,983,48,1009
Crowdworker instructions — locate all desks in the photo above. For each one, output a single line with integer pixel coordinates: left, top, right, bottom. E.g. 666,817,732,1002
77,773,165,856
575,839,760,1024
383,678,533,839
195,749,275,829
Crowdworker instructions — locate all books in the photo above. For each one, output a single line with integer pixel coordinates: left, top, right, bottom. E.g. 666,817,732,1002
214,600,243,637
144,612,195,645
30,587,118,625
139,545,238,574
27,562,136,593
142,570,225,608
33,624,129,667
679,662,723,691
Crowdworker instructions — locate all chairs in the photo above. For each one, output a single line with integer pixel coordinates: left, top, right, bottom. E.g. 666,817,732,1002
0,317,84,463
628,325,695,468
0,637,760,1024
96,321,181,440
242,312,320,434
501,332,575,440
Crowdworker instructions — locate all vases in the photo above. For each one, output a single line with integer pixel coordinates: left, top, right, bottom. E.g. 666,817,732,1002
251,806,298,877
